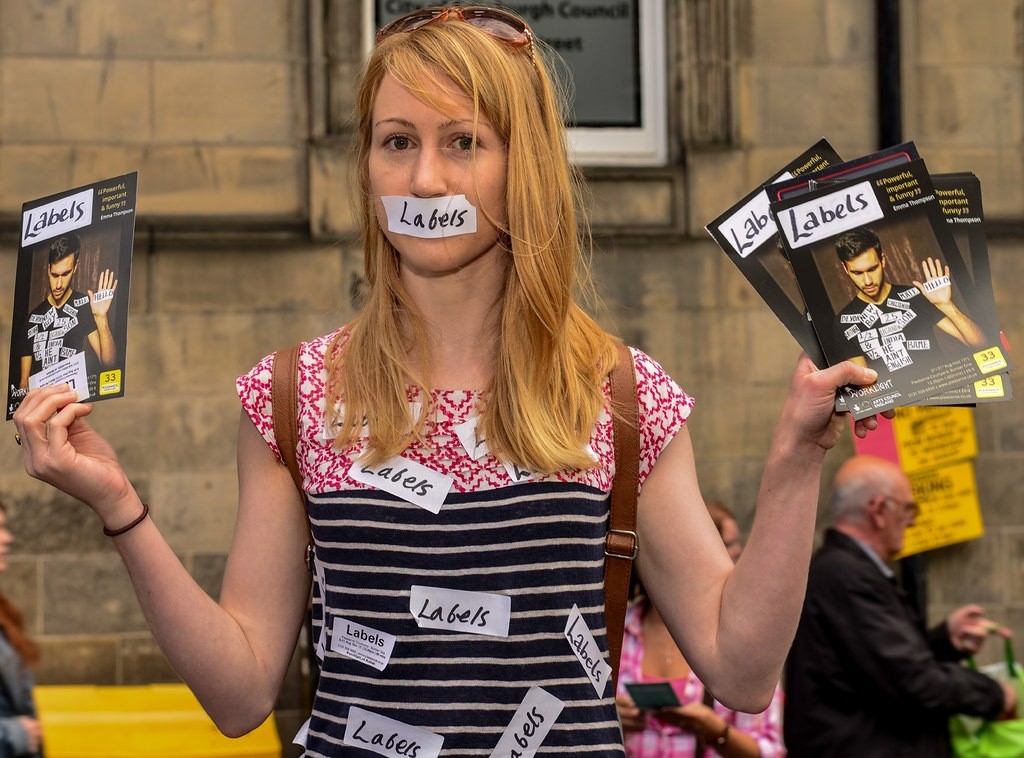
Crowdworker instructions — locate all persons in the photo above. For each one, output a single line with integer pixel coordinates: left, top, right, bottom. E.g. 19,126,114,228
12,4,896,758
833,227,988,391
20,235,117,389
0,501,47,758
615,454,1018,758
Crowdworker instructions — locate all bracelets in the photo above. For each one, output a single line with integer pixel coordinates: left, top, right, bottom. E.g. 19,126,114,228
103,504,149,537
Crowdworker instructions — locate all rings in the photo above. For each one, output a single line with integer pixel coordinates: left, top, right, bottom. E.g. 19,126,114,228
15,433,21,445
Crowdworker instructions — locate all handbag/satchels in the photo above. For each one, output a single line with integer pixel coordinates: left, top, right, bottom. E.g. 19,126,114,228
949,624,1024,757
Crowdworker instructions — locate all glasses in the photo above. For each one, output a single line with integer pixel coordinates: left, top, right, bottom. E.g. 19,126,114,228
887,497,923,519
374,6,538,67
